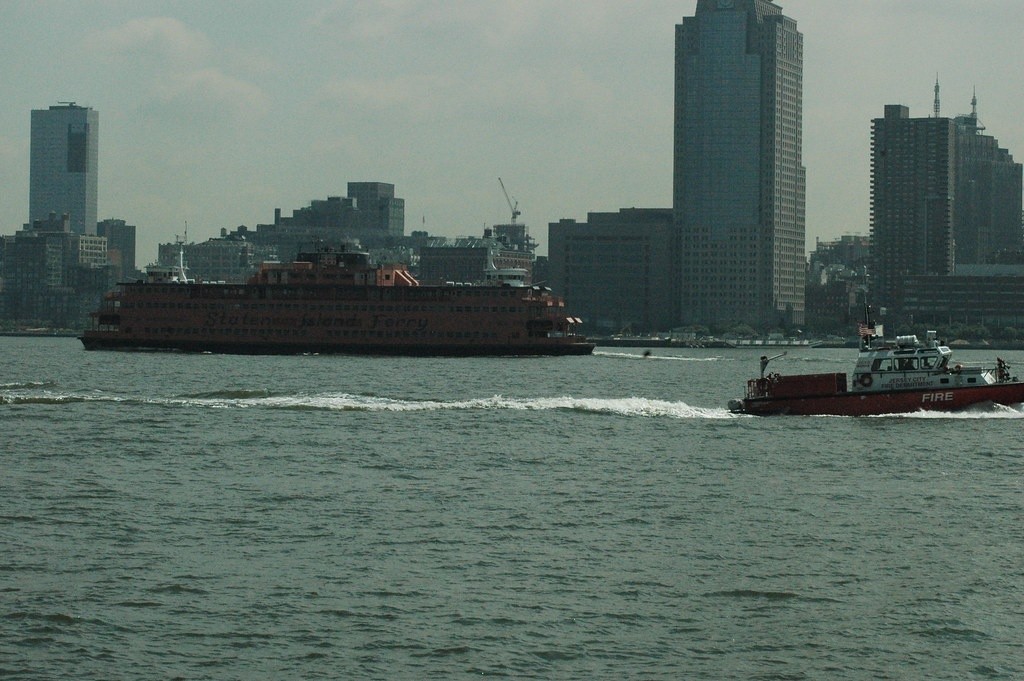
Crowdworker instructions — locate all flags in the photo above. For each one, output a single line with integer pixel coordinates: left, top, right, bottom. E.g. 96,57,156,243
857,322,876,335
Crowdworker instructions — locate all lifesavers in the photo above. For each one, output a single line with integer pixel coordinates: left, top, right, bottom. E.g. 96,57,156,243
859,375,873,386
756,377,773,393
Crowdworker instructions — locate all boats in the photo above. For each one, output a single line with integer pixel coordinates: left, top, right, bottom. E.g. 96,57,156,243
77,247,597,357
727,330,1024,416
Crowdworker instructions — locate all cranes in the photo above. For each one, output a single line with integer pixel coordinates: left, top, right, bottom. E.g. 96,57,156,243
498,177,521,224
